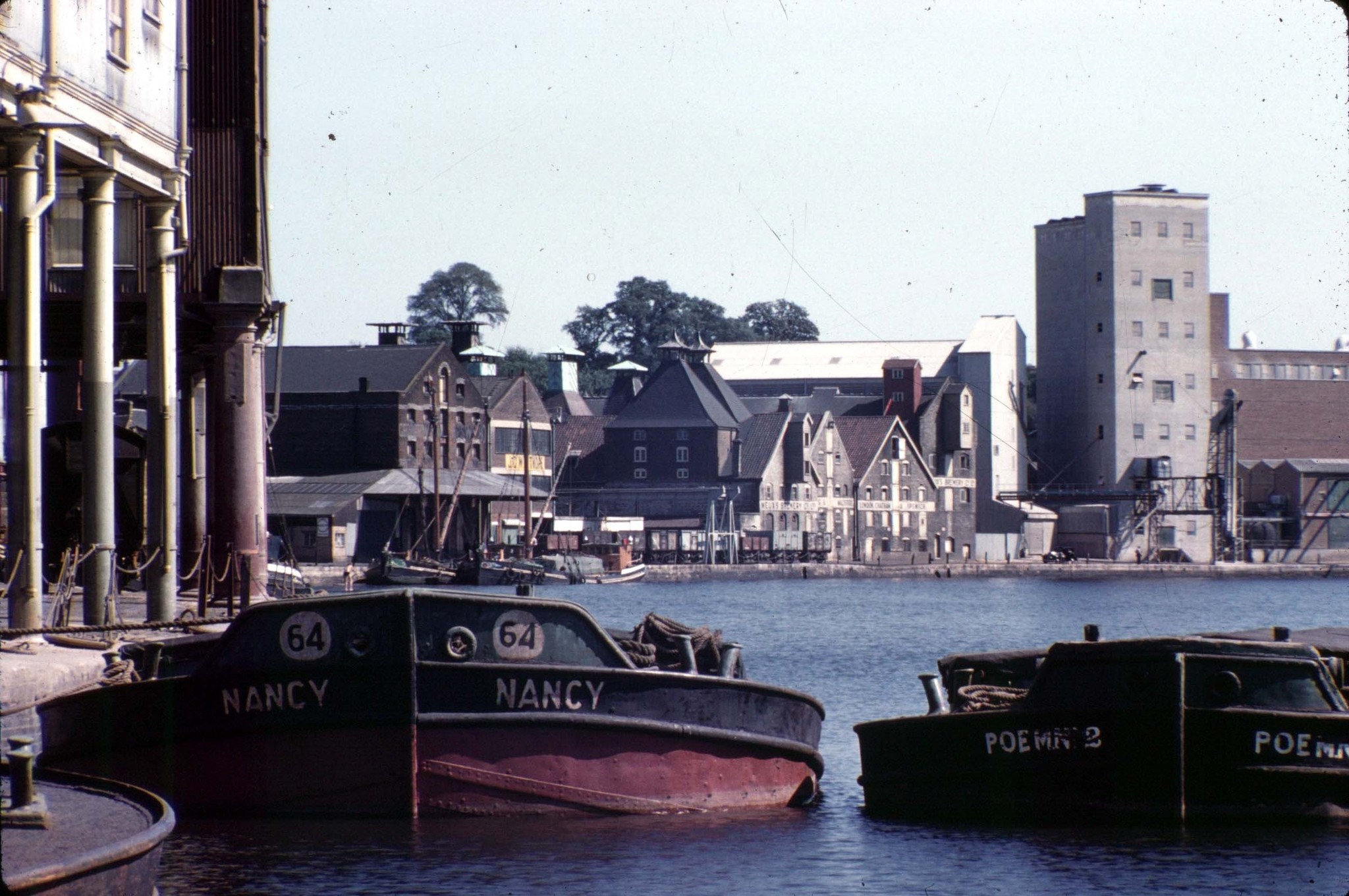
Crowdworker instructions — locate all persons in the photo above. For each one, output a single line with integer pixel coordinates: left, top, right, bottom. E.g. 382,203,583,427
478,542,488,560
344,563,359,592
1136,546,1142,564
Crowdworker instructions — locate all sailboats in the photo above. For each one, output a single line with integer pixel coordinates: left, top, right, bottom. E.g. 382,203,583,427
239,362,652,598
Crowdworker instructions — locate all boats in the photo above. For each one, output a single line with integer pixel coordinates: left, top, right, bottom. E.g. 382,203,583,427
27,575,833,829
851,618,1349,842
